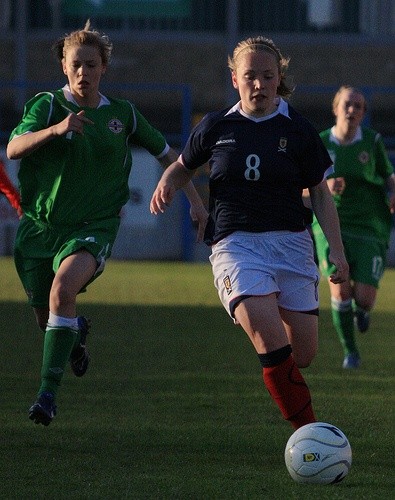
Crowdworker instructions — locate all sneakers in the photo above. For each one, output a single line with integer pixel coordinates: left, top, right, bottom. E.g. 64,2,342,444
69,314,91,377
354,309,370,334
343,352,360,369
27,391,58,426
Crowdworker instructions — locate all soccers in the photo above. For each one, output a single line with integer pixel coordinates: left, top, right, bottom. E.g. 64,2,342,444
284,421,353,486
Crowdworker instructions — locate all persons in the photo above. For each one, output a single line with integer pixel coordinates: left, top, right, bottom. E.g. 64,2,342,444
0,158,24,220
304,86,395,370
151,37,351,431
7,29,208,426
